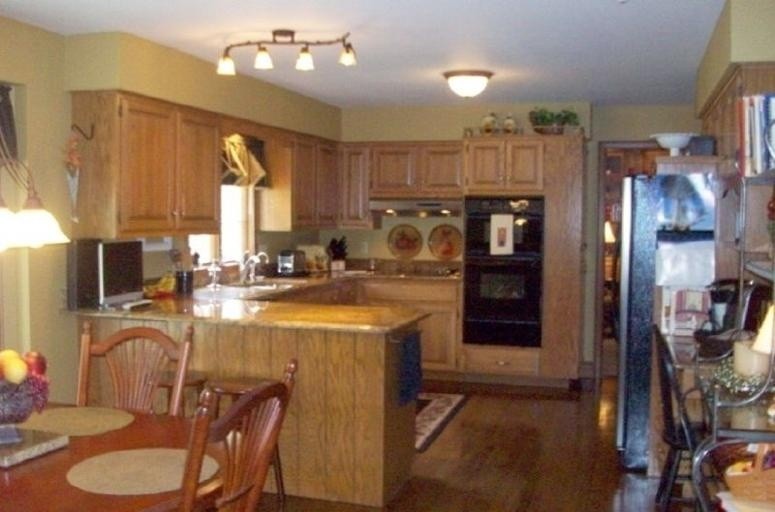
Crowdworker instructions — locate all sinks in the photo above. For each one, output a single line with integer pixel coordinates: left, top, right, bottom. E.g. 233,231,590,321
231,281,299,290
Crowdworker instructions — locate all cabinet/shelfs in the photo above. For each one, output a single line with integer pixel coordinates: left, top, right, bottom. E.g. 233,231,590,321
714,173,775,331
420,139,463,197
69,90,221,241
702,65,774,158
599,139,672,336
363,278,458,371
340,142,370,227
370,142,420,198
464,137,545,198
264,131,340,230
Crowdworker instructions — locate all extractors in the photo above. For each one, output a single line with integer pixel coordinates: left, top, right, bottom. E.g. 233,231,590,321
364,197,464,220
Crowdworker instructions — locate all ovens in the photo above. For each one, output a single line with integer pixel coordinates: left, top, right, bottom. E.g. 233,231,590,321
463,194,544,346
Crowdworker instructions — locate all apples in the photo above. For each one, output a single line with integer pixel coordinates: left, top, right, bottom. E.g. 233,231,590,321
0,349,47,384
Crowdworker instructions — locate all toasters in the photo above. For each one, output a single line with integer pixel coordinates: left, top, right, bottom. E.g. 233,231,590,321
277,249,305,274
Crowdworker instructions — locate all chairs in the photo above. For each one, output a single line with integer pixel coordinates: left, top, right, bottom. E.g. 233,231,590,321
180,357,296,512
75,319,192,414
602,240,619,344
646,322,707,508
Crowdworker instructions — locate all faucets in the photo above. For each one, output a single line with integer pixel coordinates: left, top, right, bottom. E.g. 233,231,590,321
240,256,259,285
251,251,269,283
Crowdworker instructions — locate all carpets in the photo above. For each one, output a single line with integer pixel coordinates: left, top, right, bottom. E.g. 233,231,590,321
412,391,472,451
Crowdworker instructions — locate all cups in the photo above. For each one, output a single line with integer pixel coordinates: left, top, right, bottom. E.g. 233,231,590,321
174,272,193,298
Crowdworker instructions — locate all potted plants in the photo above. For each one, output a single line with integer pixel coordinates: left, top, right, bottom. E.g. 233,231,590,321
528,105,580,135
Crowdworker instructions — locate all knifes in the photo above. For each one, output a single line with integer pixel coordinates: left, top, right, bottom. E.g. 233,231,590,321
327,236,348,260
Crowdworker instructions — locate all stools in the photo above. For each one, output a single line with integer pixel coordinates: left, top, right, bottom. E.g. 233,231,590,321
206,372,290,507
142,365,203,416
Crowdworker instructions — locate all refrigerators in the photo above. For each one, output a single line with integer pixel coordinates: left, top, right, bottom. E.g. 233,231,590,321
597,140,718,470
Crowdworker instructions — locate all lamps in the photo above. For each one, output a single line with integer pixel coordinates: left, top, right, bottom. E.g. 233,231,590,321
1,128,71,256
445,69,489,98
216,30,355,77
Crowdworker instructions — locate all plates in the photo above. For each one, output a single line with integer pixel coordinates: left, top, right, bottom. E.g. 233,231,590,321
387,224,422,260
427,224,463,260
